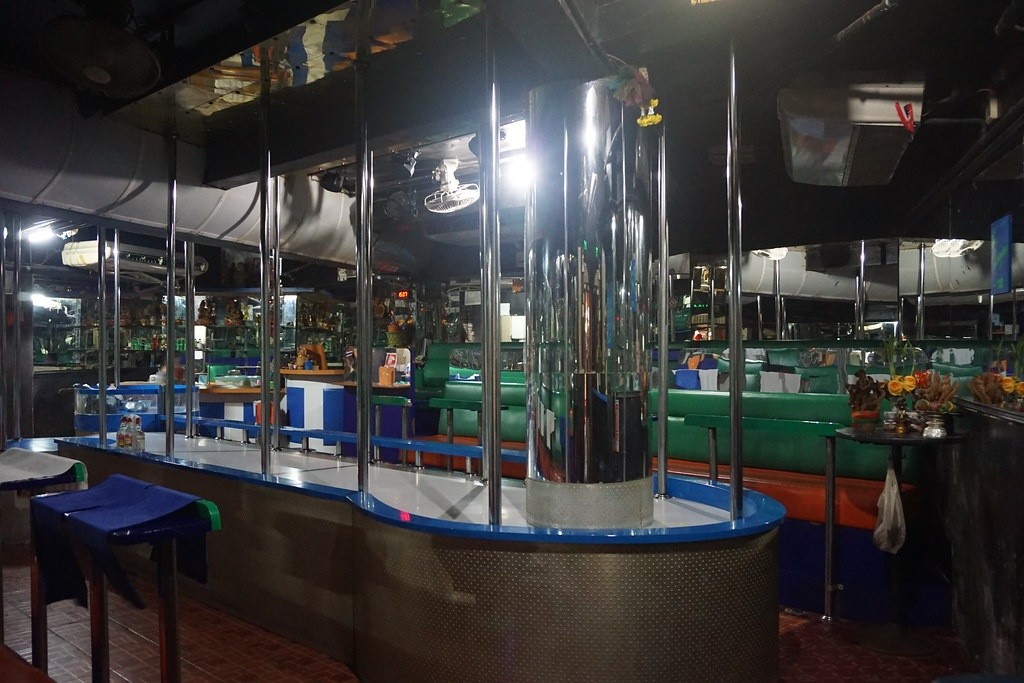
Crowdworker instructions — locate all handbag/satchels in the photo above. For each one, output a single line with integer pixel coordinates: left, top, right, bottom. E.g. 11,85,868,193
872,459,906,553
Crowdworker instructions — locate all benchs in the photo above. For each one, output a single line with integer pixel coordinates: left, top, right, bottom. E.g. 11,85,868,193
400,340,1024,626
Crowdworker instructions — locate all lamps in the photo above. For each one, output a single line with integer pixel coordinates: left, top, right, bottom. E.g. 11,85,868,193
400,147,418,177
320,172,345,192
384,190,416,220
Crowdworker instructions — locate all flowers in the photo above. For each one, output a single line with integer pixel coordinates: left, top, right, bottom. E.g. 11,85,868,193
985,338,1024,414
878,332,932,399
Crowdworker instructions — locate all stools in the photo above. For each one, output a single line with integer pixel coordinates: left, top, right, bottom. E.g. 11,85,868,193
0,446,85,683
31,474,222,683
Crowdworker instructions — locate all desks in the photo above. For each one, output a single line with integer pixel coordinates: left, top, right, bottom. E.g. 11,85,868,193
836,428,969,654
199,369,410,461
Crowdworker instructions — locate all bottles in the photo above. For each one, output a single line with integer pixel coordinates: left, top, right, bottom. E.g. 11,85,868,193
312,361,319,370
116,417,145,454
132,334,187,351
305,359,313,370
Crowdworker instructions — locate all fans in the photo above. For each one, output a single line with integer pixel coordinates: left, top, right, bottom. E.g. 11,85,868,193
424,158,480,213
